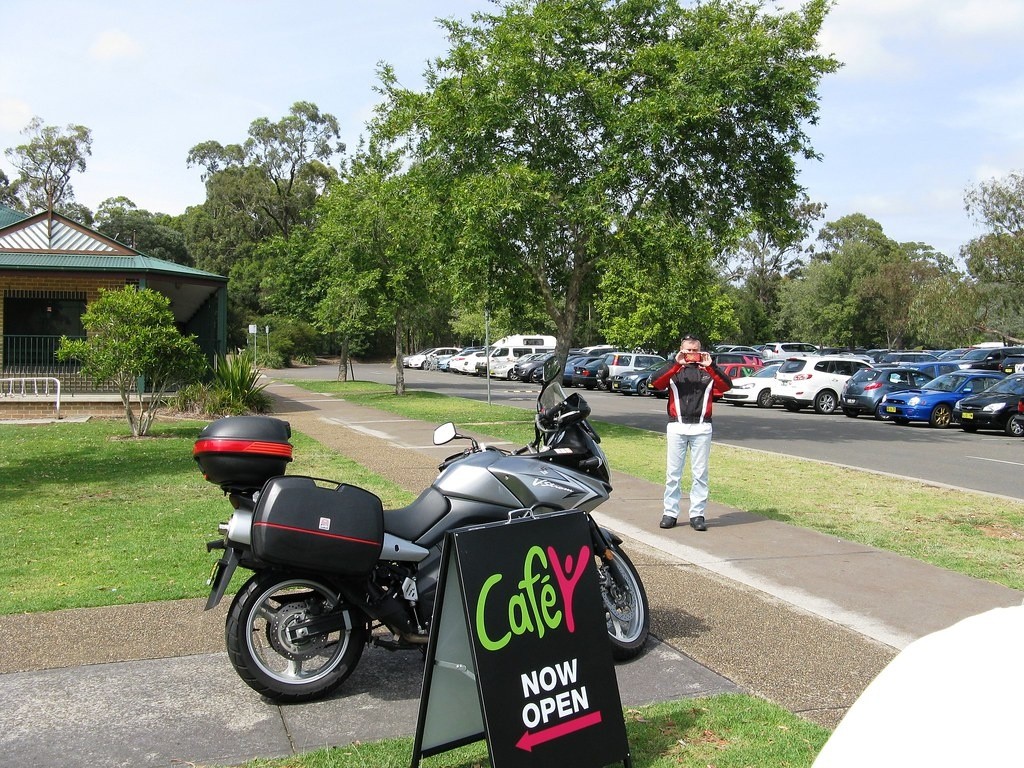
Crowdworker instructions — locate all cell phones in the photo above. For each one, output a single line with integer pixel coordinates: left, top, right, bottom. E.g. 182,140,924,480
685,353,701,362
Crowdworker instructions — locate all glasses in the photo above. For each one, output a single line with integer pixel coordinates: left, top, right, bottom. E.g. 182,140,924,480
682,335,702,347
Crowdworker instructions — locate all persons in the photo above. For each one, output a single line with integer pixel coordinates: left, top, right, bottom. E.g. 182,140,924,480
645,334,733,531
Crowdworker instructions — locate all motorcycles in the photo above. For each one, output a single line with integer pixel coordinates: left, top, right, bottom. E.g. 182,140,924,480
190,356,652,704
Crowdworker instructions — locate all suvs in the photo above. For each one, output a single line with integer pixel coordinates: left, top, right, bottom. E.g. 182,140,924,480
770,354,882,415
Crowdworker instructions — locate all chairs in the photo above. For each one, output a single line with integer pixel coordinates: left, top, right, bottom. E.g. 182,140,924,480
897,376,908,384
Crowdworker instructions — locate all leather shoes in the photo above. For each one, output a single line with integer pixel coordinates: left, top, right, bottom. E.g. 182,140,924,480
660,515,677,528
690,516,707,531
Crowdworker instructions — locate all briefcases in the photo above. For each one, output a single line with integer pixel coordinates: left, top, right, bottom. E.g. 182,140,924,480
250,475,385,583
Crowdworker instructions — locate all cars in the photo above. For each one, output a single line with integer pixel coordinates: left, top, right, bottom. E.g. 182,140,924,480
839,365,955,421
951,371,1024,437
880,368,1023,429
401,331,1024,402
721,363,838,409
